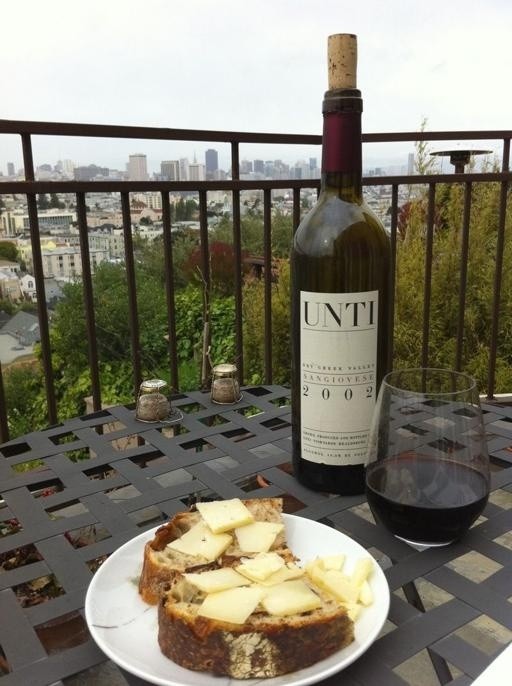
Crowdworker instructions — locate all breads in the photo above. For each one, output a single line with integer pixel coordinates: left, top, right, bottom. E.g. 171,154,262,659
142,496,354,679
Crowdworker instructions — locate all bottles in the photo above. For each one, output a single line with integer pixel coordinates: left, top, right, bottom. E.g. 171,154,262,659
286,33,392,501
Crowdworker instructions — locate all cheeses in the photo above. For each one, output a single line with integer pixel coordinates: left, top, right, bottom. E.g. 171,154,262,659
167,499,321,622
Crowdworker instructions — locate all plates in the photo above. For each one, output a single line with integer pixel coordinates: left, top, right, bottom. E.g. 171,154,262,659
86,508,390,686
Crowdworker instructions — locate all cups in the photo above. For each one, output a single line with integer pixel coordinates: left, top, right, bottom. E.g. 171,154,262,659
361,367,494,547
